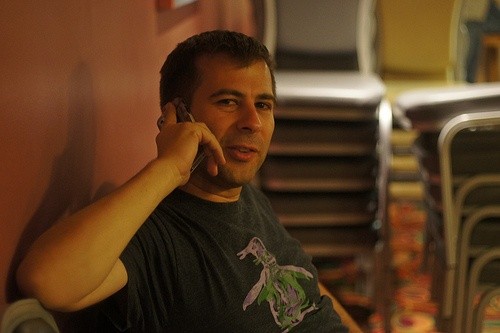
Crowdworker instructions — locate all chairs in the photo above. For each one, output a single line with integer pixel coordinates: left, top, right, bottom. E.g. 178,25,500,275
0,0,500,333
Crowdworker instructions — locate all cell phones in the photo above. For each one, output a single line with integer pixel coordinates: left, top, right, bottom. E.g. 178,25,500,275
157,97,207,173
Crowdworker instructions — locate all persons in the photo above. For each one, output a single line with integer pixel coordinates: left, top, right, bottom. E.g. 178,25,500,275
13,29,365,333
